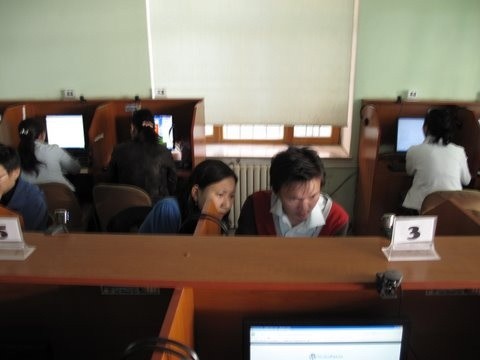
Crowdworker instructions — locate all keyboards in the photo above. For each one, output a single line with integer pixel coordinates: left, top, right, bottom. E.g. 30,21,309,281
387,162,406,172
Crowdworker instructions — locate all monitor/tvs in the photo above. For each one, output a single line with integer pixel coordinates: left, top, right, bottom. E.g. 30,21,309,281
130,114,175,151
395,115,428,154
42,113,86,153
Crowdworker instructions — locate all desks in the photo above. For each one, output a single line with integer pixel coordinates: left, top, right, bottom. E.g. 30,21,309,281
376,158,407,176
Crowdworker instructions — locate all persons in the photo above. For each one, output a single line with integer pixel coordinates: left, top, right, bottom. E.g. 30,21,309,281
137,160,238,235
16,118,81,192
391,109,472,216
235,147,351,236
0,143,54,233
104,109,177,202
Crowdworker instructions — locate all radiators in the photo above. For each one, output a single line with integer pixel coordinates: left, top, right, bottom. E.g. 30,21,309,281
229,163,270,229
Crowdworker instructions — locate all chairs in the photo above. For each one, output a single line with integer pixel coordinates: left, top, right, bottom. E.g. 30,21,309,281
39,183,74,213
93,184,152,230
